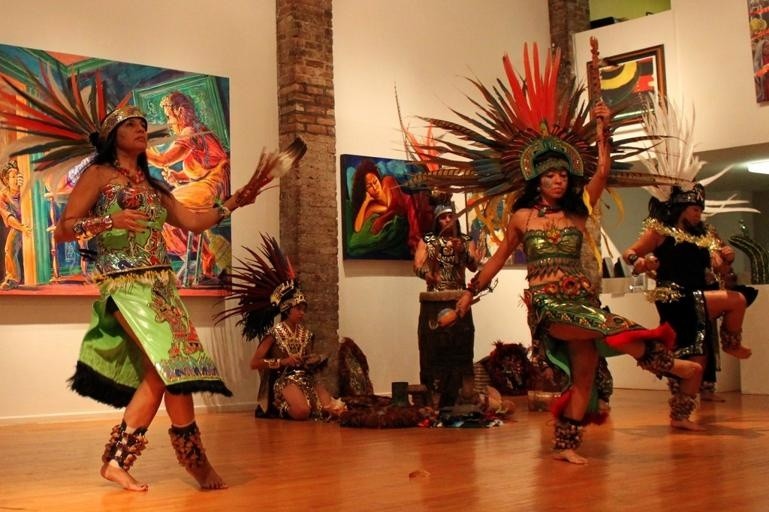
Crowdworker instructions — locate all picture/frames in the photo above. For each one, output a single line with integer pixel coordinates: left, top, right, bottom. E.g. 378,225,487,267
587,44,668,128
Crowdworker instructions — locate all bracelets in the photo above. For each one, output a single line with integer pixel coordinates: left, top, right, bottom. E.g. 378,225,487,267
217,203,231,221
73,214,113,240
264,359,281,370
721,257,735,265
628,253,639,264
464,271,492,296
597,132,610,143
415,267,430,279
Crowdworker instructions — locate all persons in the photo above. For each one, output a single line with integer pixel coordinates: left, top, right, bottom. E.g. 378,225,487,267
0,159,34,292
700,226,740,403
414,200,494,428
621,186,751,433
52,108,261,493
454,99,704,466
351,160,435,254
146,89,230,284
250,286,332,420
